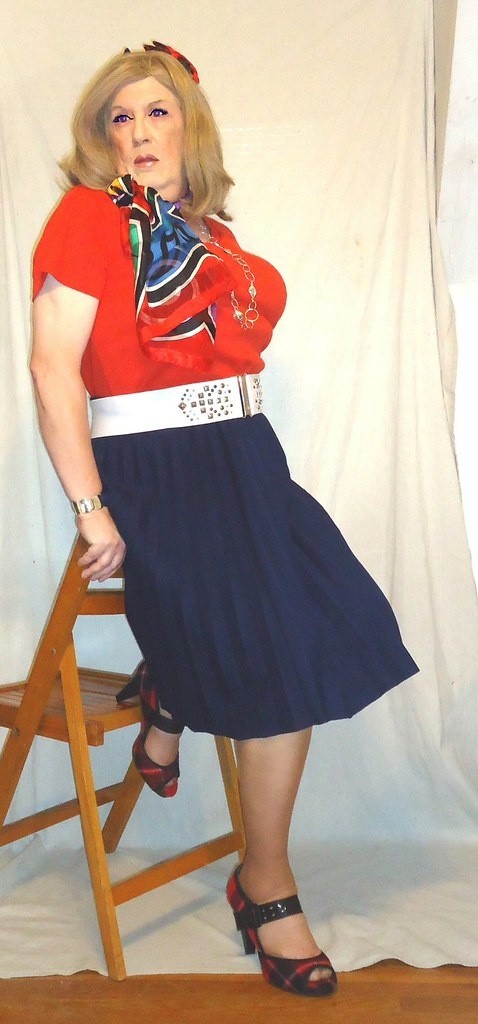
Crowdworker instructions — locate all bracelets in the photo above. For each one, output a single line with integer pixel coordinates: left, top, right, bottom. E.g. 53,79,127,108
70,496,105,514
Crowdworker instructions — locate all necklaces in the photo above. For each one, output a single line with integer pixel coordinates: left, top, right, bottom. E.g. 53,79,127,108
198,221,259,332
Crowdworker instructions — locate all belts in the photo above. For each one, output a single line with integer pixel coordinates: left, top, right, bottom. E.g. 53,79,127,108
90,372,263,439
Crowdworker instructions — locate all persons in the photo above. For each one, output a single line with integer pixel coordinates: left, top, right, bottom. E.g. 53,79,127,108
26,38,419,996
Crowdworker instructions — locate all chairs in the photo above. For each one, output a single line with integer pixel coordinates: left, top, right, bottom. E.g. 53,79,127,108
0,533,247,982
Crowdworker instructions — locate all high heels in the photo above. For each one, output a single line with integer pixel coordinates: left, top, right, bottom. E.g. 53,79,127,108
224,865,336,995
115,657,185,797
